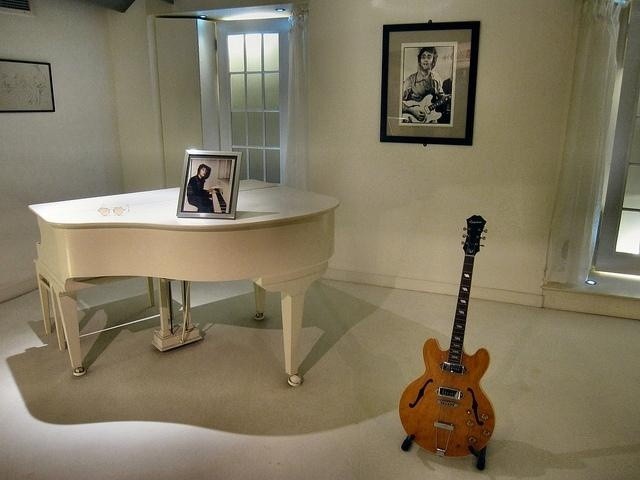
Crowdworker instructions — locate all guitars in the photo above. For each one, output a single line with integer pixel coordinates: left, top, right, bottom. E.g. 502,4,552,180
399,216,496,458
402,94,451,123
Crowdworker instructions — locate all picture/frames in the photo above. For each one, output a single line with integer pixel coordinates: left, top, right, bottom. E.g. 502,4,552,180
176,150,242,219
380,20,481,147
1,59,55,113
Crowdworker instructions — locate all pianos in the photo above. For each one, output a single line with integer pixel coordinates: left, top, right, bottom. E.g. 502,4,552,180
209,186,227,213
29,179,339,387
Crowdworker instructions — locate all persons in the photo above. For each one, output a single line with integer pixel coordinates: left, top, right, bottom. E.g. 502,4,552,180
401,47,452,125
185,164,216,214
